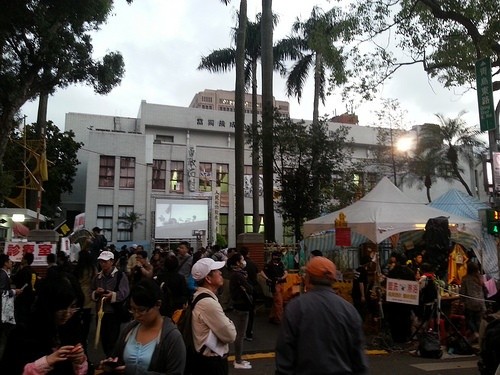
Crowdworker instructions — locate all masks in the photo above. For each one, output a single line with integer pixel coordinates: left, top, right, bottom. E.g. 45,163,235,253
240,260,247,269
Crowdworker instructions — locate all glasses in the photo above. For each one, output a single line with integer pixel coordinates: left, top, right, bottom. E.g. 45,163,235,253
57,306,81,313
135,256,145,259
127,305,152,314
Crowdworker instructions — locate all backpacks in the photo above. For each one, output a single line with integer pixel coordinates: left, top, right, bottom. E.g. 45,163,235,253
173,291,218,358
219,272,237,312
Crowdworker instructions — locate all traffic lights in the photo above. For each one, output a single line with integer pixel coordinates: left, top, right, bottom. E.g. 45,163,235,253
486,208,500,237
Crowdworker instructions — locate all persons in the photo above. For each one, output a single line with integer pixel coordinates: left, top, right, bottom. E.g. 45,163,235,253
311,250,322,258
353,253,500,375
275,256,368,375
0,227,287,375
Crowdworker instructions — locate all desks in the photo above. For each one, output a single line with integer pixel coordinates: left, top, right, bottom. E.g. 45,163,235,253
441,292,462,332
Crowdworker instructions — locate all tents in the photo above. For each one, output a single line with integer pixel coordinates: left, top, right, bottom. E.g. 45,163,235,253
303,177,484,283
428,187,500,281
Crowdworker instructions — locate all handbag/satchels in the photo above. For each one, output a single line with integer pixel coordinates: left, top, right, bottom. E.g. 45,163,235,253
1,289,16,324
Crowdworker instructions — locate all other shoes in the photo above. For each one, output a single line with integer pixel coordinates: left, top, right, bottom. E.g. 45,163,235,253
233,360,252,369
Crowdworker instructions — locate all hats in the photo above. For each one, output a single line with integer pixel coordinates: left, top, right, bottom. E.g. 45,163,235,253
97,251,114,261
300,256,337,280
191,258,225,280
